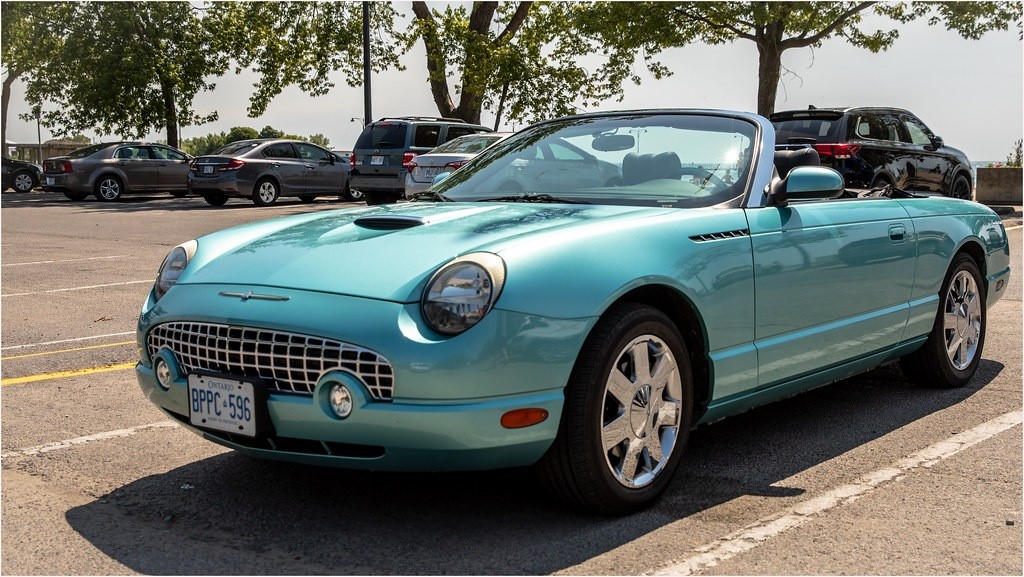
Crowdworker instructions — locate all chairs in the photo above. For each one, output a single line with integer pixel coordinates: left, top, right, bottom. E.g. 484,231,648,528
620,152,682,186
124,149,133,159
774,148,820,183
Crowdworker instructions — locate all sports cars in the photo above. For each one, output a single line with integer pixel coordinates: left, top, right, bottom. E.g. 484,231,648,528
135,105,1011,514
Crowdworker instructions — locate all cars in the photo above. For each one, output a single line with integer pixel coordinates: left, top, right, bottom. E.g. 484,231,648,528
185,138,364,208
40,140,195,201
2,156,43,193
403,131,622,196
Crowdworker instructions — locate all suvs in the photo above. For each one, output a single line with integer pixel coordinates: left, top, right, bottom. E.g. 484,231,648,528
767,106,977,199
349,116,496,207
